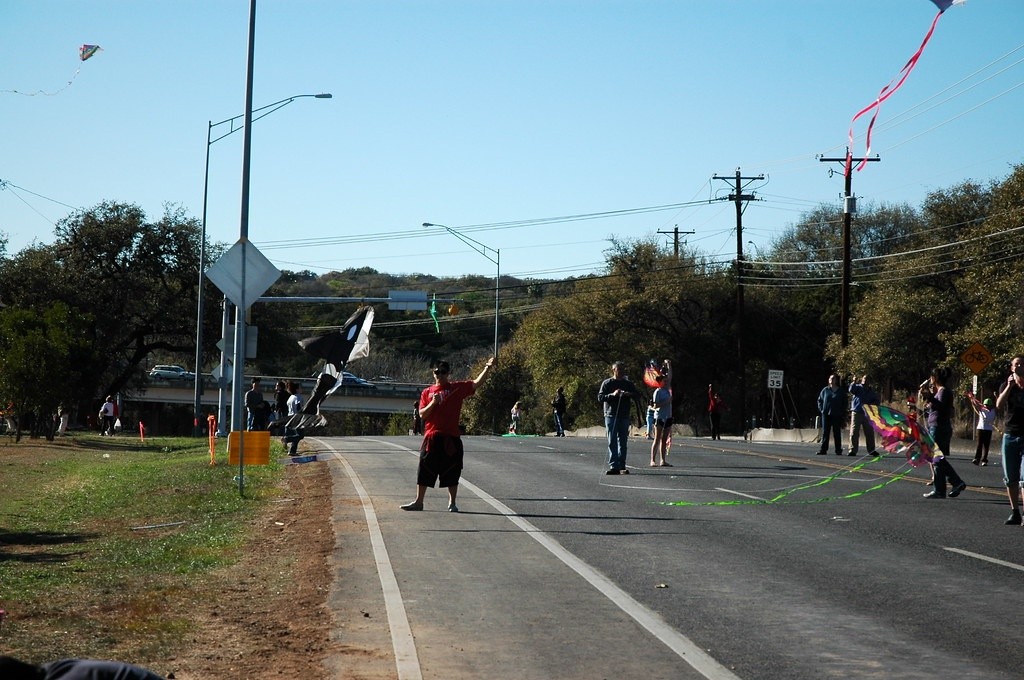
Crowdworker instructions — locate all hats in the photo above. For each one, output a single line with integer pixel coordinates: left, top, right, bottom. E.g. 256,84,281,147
434,360,450,372
984,398,992,405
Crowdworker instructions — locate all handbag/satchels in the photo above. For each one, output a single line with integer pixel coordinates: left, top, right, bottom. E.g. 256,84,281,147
103,403,108,413
114,417,122,432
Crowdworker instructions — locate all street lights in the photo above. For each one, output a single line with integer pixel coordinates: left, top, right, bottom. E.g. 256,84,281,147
192,93,333,437
422,222,500,436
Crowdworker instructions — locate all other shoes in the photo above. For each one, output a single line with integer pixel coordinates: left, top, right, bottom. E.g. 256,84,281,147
972,459,979,465
448,504,458,512
870,451,879,456
287,452,298,456
815,450,827,455
982,462,986,466
1004,513,1024,526
401,502,424,510
927,480,934,486
837,451,842,455
847,451,856,456
619,470,629,475
281,437,287,449
606,469,620,475
660,461,673,467
651,462,658,466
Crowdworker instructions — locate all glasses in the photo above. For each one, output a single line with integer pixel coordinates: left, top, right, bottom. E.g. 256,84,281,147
434,370,447,375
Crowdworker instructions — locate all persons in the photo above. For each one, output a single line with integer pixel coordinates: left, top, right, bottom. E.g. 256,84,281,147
401,356,497,513
413,401,421,435
0,400,18,434
646,399,655,440
849,375,880,456
56,403,71,436
597,360,642,475
510,401,522,434
245,377,304,454
99,395,118,437
651,374,672,467
817,373,848,456
995,354,1024,527
708,384,721,440
551,387,566,437
972,398,995,466
919,367,965,499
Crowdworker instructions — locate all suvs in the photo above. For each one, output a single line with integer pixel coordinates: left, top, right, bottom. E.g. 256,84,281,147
149,364,194,382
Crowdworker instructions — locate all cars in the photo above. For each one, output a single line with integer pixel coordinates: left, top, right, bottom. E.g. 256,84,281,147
342,369,396,390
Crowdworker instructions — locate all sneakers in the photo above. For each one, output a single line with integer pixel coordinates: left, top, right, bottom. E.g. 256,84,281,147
923,491,946,499
948,483,966,497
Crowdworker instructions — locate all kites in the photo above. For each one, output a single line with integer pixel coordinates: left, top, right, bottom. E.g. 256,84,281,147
286,304,375,441
644,366,663,388
839,404,944,500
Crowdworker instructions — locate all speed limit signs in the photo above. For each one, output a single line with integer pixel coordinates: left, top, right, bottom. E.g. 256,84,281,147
767,370,784,389
